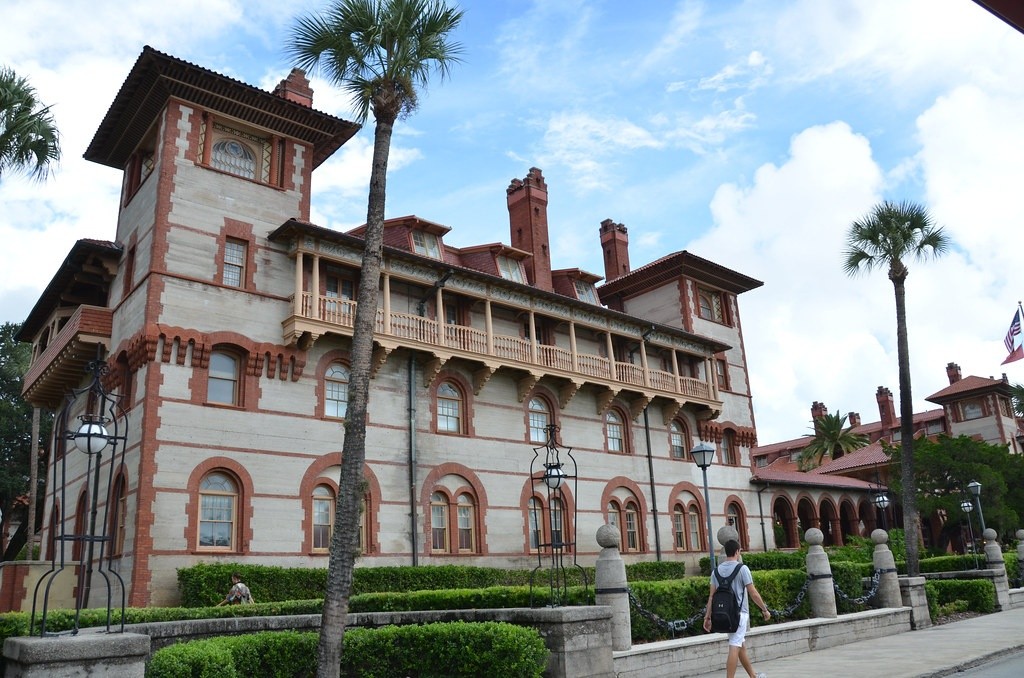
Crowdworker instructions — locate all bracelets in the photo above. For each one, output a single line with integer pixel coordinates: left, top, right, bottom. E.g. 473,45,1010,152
704,617,711,620
763,609,768,612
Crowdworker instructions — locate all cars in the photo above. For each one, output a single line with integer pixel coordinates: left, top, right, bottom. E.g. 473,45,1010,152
966,538,981,553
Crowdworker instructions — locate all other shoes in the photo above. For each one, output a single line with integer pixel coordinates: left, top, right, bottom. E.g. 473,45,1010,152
756,672,766,678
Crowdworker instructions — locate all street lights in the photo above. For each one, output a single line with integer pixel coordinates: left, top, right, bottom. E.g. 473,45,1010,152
31,342,126,635
690,444,716,570
957,491,991,569
868,479,900,555
530,424,588,608
967,479,986,531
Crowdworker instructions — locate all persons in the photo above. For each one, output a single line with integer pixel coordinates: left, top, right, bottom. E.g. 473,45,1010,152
217,572,254,606
703,539,770,678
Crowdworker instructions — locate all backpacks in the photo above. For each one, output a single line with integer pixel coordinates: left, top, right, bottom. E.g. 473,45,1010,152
710,563,746,633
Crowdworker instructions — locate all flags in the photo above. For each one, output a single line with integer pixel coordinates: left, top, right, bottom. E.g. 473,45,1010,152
1004,307,1024,355
